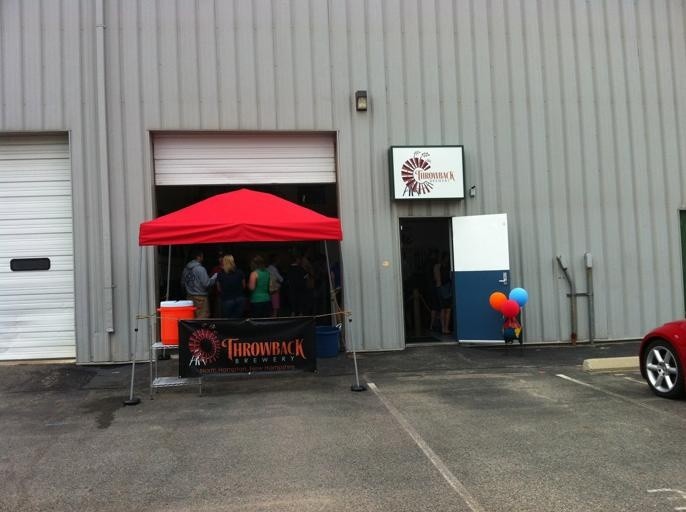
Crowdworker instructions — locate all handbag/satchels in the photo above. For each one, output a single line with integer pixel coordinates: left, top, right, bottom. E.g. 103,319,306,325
268,271,281,295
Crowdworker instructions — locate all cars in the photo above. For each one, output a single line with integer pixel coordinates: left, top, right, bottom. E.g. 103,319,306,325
636,320,685,403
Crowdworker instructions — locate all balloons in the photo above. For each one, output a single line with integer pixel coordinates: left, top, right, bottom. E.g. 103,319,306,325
488,287,530,328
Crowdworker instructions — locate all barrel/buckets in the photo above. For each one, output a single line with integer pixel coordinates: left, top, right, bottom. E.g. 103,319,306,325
158,301,197,346
314,326,340,357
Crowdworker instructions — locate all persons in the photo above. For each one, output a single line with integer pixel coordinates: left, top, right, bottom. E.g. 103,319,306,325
172,243,453,335
